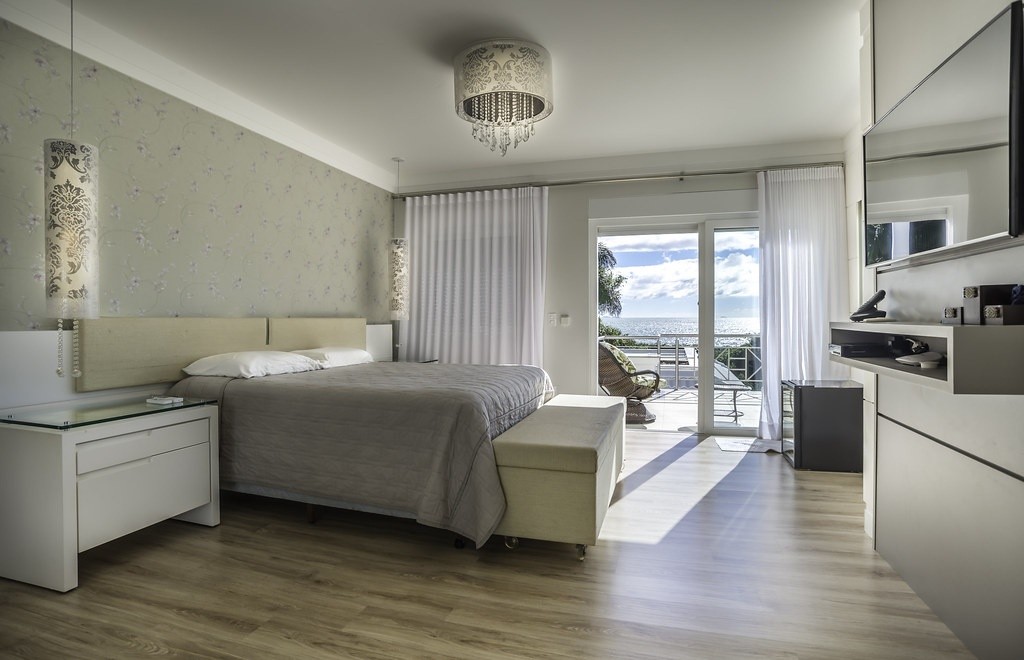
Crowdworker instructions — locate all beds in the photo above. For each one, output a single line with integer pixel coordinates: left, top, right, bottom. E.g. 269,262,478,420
75,315,557,559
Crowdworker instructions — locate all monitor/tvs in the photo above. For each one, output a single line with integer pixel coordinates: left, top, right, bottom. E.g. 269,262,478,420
863,0,1023,268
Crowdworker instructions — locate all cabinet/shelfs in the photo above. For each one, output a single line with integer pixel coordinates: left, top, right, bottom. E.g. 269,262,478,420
826,318,1024,660
781,378,864,472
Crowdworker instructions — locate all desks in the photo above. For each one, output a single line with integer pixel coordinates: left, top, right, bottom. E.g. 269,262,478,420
695,383,752,427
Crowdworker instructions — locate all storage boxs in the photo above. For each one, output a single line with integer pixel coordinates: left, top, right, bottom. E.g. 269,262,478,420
963,284,1017,325
984,305,1024,325
941,307,964,325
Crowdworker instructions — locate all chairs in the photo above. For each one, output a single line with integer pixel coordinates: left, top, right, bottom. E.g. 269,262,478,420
598,342,668,423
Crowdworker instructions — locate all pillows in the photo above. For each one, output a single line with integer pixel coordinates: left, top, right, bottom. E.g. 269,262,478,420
289,347,376,369
181,349,324,379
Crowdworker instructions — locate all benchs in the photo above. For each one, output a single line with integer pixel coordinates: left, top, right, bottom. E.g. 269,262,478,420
491,394,628,563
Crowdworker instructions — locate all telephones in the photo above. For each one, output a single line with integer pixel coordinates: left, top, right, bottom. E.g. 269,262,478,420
850,290,887,322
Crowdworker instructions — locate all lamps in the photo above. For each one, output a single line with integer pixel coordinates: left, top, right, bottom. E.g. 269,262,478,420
452,37,554,157
389,238,408,348
44,136,101,378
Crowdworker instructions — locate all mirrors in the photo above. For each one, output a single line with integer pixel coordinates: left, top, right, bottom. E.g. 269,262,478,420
862,0,1024,126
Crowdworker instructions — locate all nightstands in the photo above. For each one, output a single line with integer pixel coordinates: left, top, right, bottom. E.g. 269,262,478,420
0,394,221,593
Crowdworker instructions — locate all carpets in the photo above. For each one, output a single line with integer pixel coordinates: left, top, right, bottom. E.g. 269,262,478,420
714,436,781,454
781,439,795,454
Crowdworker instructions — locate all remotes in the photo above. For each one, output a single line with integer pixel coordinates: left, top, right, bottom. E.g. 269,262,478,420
146,396,184,404
